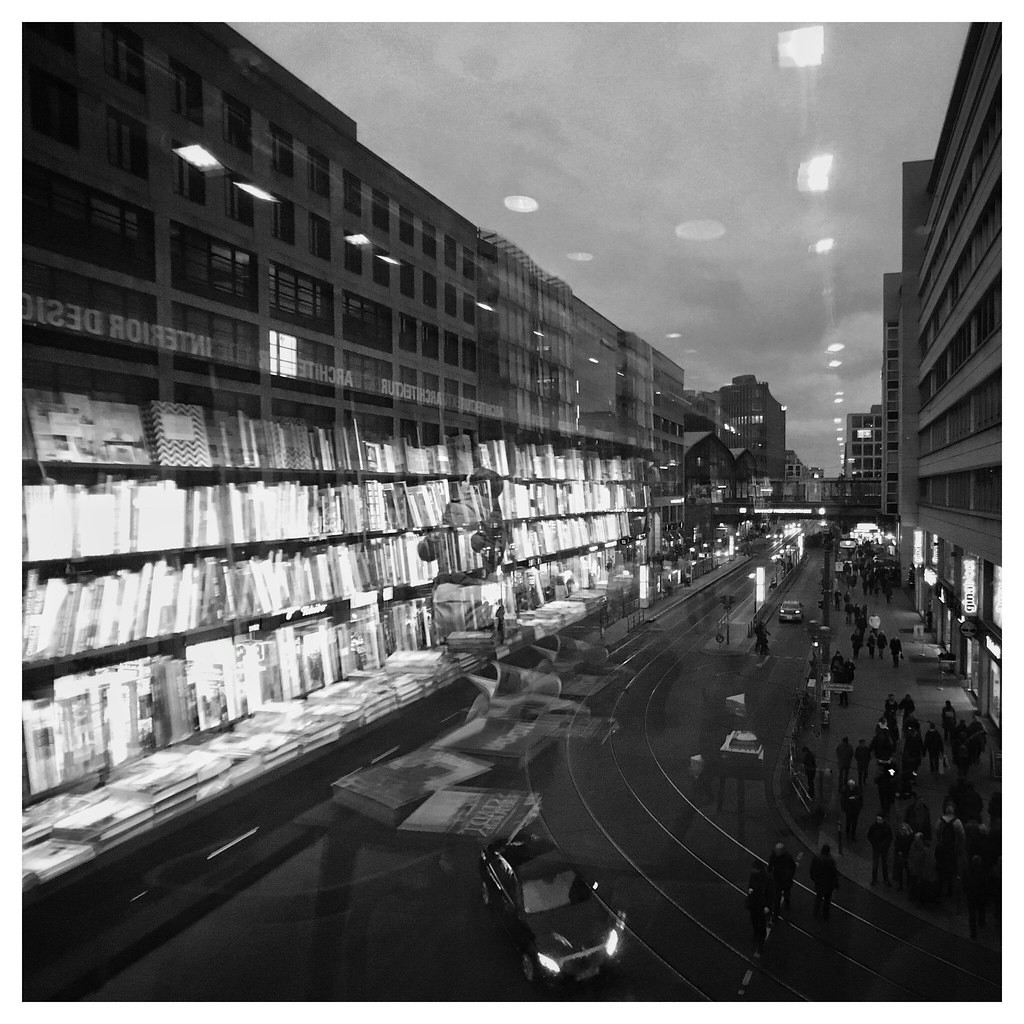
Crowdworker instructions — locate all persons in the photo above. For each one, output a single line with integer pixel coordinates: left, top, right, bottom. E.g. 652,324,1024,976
810,844,840,922
837,737,853,793
665,533,730,571
767,843,797,923
876,630,888,661
845,600,854,625
801,746,817,798
834,590,842,612
745,861,774,953
779,554,785,570
853,604,860,624
768,578,777,592
851,629,863,659
868,612,880,639
861,604,868,617
835,541,893,606
841,779,864,843
664,580,673,596
868,814,893,887
856,614,868,638
866,631,876,659
873,691,1002,946
889,636,903,668
830,650,855,710
683,574,691,587
855,740,872,788
753,617,773,656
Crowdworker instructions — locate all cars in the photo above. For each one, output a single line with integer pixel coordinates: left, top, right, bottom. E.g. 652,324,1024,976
480,835,623,990
778,600,804,622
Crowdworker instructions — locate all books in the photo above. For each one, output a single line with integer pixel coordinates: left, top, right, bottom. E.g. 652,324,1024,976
22,383,665,898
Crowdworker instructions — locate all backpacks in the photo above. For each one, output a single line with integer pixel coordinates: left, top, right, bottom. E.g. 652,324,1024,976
939,816,958,844
